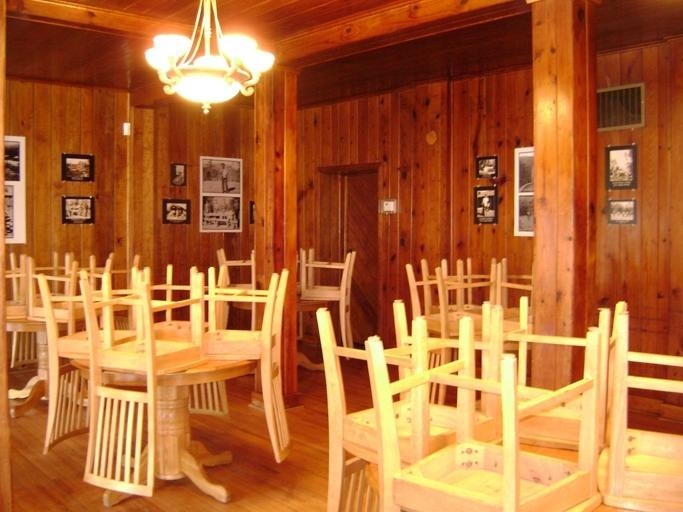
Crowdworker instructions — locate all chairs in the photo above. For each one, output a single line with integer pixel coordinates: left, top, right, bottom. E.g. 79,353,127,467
4,249,683,512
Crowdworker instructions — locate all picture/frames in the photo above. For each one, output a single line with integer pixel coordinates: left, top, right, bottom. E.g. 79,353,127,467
475,156,496,177
199,155,243,233
473,187,495,224
162,198,190,223
61,153,95,182
171,163,186,186
4,135,26,244
514,146,536,238
608,200,635,224
61,195,93,223
604,145,636,191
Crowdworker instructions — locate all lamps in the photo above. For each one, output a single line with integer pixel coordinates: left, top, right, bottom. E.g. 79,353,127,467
146,0,273,117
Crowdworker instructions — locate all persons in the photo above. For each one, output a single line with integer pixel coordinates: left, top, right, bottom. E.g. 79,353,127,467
166,205,187,219
219,163,229,193
481,194,490,210
